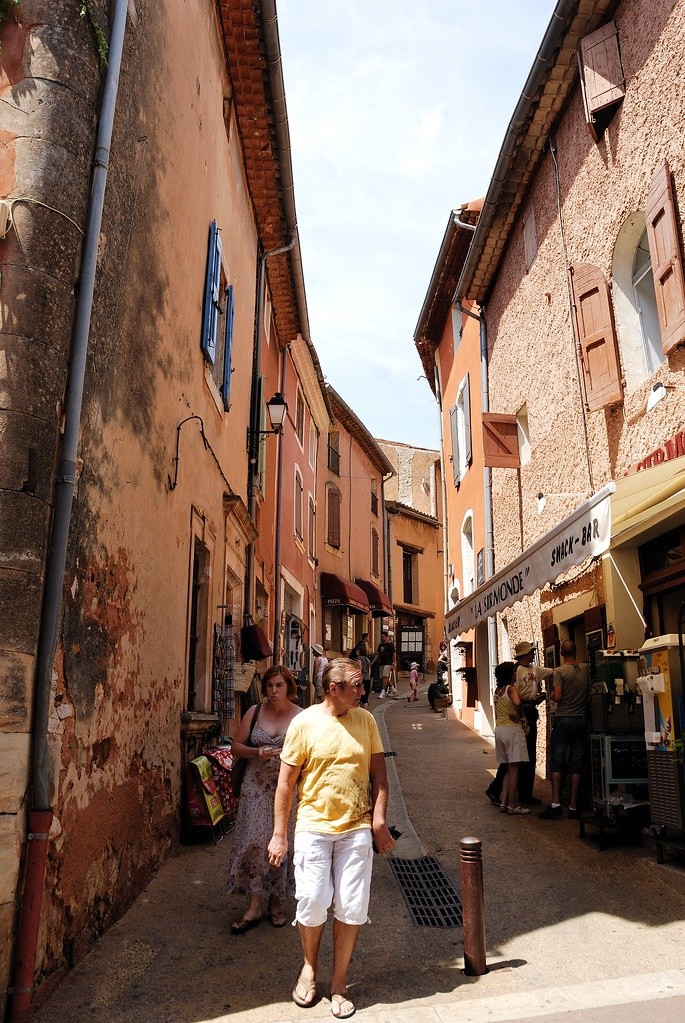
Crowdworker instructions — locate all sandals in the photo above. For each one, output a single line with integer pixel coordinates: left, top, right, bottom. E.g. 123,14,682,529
508,805,531,815
499,804,507,813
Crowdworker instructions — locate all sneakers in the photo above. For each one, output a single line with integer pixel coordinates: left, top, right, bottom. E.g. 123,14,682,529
378,692,386,699
389,689,398,697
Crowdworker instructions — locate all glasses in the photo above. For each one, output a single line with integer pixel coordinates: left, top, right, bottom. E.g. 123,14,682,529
327,682,364,691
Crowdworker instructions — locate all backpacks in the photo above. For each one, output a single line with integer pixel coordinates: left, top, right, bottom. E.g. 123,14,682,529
358,640,366,655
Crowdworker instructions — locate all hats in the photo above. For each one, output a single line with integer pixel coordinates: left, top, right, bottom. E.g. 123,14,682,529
410,661,420,670
513,641,537,659
312,644,324,656
438,642,447,647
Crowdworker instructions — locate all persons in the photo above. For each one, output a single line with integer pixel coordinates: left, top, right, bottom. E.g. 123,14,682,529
407,662,421,701
229,666,303,934
428,641,448,708
311,643,329,703
485,642,554,805
539,641,589,818
349,632,397,709
493,662,532,813
268,658,395,1019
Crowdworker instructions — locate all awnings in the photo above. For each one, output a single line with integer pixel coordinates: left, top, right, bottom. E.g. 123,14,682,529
355,578,394,619
321,572,368,614
445,453,684,640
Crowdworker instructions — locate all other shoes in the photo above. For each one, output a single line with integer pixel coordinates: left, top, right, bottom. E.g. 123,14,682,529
518,796,543,806
538,803,563,819
407,695,410,702
413,697,420,701
568,809,582,819
486,789,501,806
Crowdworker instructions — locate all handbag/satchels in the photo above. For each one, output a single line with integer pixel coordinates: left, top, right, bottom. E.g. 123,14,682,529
241,613,273,661
230,752,248,797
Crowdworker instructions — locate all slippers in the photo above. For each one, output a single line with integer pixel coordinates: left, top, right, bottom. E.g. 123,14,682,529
292,962,318,1008
329,987,355,1019
232,915,261,932
267,904,286,927
